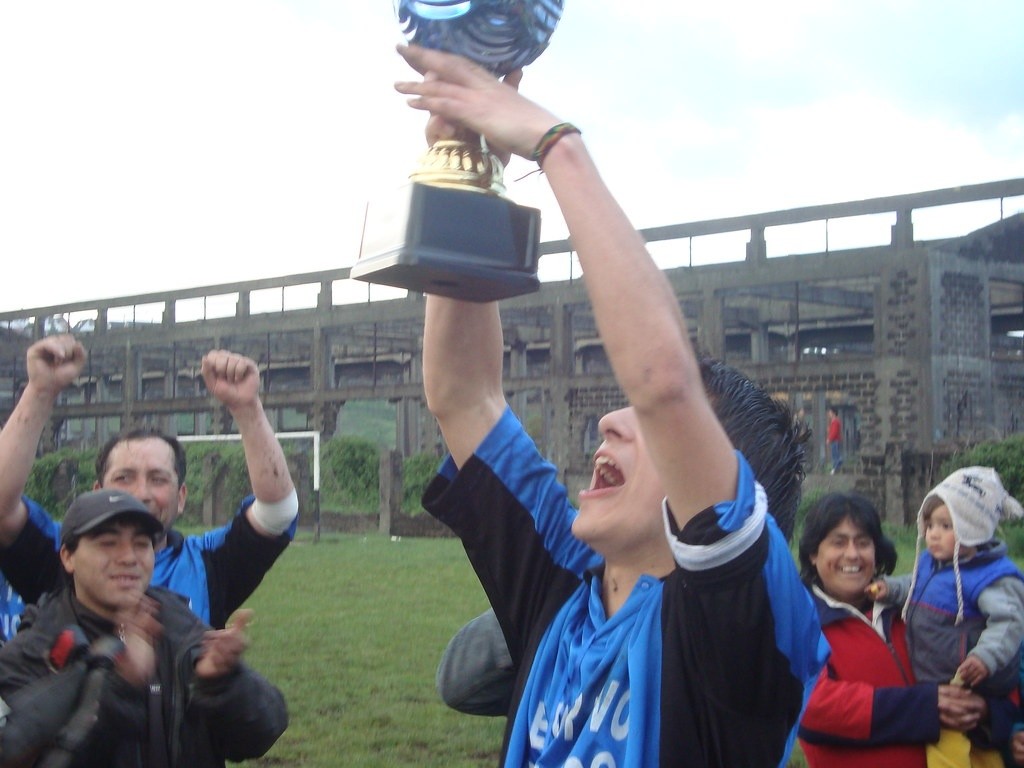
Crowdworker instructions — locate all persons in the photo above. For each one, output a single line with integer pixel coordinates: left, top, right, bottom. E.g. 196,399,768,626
0,332,300,643
0,488,288,768
826,407,843,474
394,43,828,768
793,466,1024,768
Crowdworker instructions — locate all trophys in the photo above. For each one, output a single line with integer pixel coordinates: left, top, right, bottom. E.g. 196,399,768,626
349,0,564,303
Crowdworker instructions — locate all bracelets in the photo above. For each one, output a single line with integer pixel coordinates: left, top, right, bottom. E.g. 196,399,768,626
513,123,581,183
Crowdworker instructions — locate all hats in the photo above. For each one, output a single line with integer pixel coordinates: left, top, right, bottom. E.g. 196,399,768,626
901,465,1024,627
60,489,164,550
